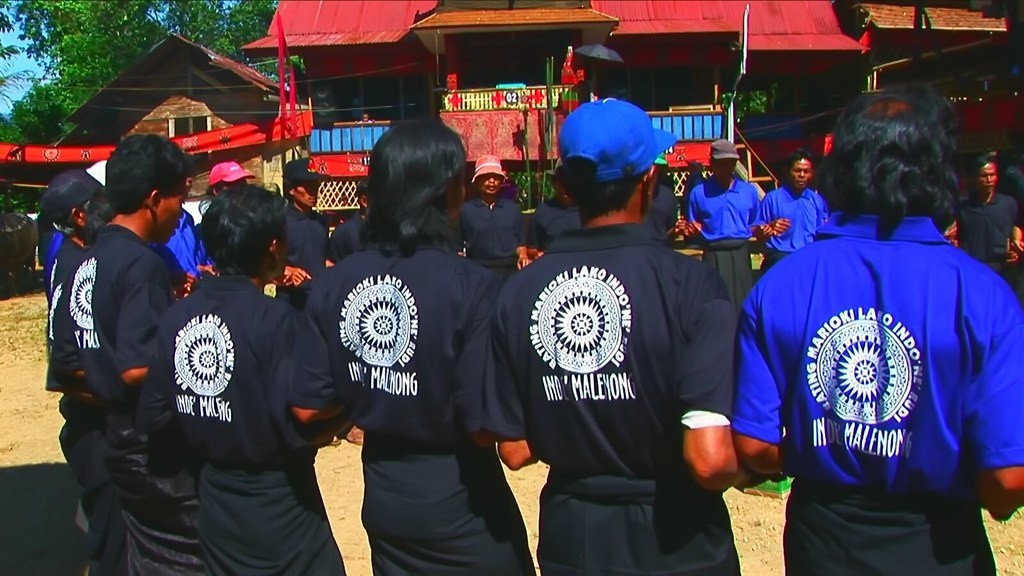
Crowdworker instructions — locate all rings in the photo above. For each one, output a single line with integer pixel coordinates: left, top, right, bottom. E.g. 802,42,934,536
775,222,779,226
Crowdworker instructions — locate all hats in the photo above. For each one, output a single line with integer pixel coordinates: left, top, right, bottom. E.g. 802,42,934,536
285,157,330,185
557,97,679,184
39,168,98,223
710,140,740,159
209,162,255,187
472,155,506,183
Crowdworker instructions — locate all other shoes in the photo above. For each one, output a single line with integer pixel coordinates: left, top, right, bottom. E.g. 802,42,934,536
346,426,365,444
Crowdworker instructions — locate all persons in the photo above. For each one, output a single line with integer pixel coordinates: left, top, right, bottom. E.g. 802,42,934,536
37,87,1024,576
